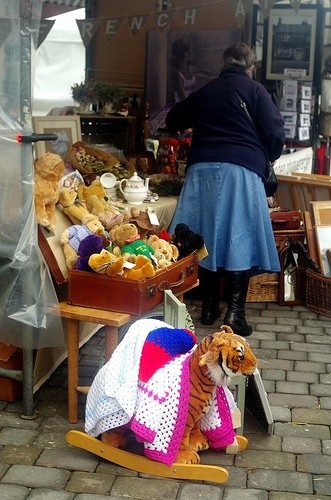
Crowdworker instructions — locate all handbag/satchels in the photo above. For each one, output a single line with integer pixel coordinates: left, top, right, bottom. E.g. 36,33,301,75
263,159,278,197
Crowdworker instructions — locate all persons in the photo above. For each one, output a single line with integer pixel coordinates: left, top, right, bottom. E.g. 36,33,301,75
164,42,287,337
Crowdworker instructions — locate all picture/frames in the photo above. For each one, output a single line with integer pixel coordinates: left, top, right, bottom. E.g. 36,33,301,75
260,4,323,141
32,115,81,160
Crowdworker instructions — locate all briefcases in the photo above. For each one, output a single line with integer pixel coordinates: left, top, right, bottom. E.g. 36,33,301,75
35,193,200,314
269,210,301,229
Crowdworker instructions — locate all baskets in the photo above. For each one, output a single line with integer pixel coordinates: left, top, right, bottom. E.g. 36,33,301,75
305,268,331,318
286,266,308,302
245,274,280,302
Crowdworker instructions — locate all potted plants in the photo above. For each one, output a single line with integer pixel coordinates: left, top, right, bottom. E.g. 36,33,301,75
70,81,96,115
95,80,126,113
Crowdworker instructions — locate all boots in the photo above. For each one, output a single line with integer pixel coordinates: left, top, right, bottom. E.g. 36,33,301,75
197,267,222,324
223,272,253,336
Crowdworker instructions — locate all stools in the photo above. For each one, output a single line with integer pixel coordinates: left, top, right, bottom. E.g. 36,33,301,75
44,279,200,424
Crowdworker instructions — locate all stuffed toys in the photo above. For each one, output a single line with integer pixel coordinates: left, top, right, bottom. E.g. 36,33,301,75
33,151,179,280
158,136,185,176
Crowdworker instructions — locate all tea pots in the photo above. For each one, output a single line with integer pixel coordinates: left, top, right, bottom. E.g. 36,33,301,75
119,172,149,205
